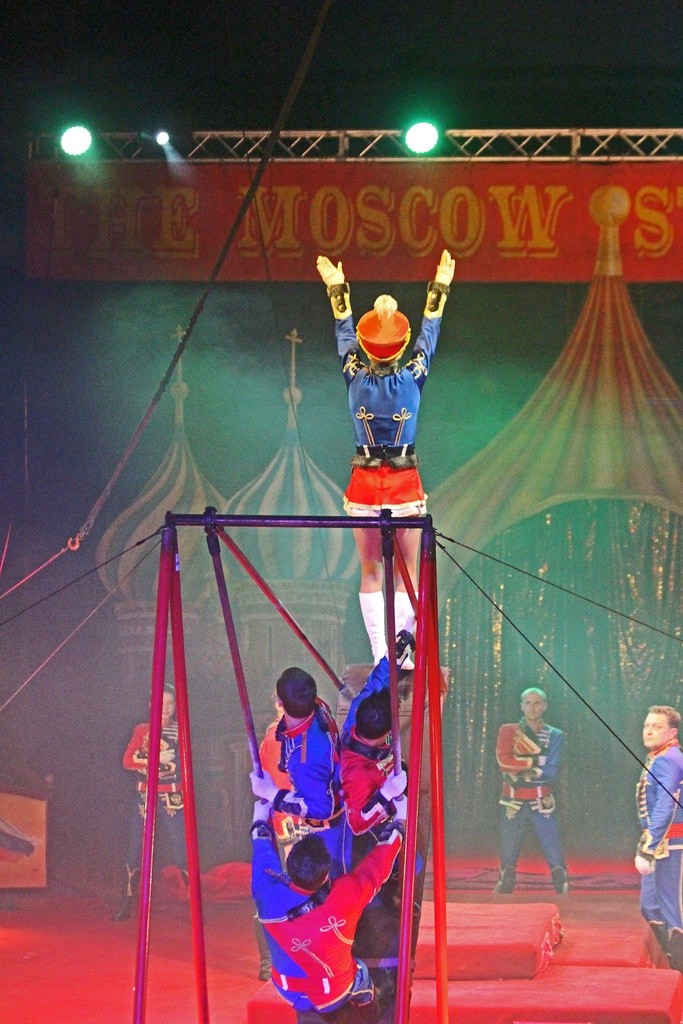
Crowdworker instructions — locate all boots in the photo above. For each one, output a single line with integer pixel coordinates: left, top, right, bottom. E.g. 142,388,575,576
182,869,188,890
551,865,569,894
496,865,523,892
113,863,139,921
649,920,667,954
254,916,271,980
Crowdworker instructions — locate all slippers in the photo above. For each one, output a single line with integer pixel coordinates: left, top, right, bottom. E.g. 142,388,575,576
667,926,683,970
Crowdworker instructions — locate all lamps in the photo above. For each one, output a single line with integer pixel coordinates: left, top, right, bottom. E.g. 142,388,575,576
139,129,170,146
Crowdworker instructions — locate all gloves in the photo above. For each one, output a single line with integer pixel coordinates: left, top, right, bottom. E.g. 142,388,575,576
380,771,407,801
249,771,278,802
253,800,273,822
317,255,345,288
393,795,408,821
405,613,417,634
435,249,456,286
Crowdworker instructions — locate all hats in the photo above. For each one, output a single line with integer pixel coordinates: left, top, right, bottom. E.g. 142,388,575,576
356,295,410,363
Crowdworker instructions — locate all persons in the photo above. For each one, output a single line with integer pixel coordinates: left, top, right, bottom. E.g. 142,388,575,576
249,611,427,1023
314,248,457,670
633,704,683,971
109,683,201,922
494,686,572,894
